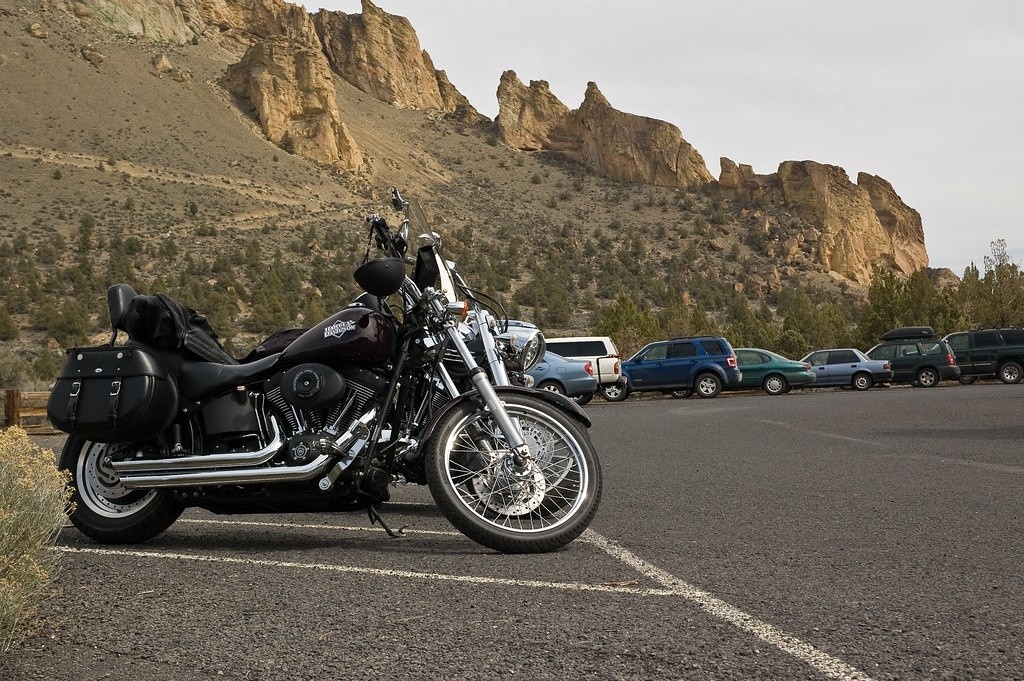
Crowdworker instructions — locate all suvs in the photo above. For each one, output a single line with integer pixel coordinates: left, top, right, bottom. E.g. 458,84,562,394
899,327,1024,388
601,335,743,402
543,337,628,405
840,326,962,391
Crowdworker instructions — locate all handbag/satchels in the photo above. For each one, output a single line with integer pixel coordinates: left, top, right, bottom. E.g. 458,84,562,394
46,345,179,444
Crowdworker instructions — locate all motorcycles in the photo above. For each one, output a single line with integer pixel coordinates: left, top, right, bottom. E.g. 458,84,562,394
47,185,604,555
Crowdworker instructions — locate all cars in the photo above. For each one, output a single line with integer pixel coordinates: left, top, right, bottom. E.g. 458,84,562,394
662,347,817,400
784,348,894,393
522,349,598,397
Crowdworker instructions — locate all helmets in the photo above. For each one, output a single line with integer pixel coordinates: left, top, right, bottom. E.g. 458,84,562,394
353,257,405,297
